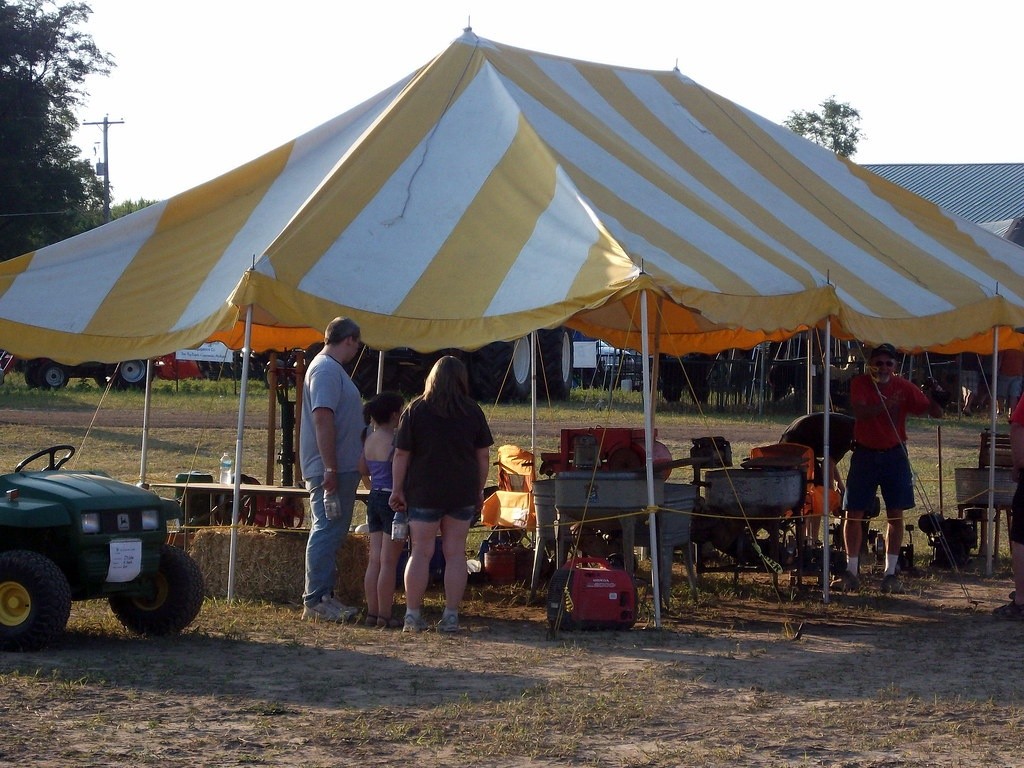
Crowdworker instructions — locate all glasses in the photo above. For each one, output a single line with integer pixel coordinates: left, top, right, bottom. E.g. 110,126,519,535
871,361,895,367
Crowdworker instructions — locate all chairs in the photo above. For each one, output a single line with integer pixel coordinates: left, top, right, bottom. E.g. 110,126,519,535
954,431,1024,562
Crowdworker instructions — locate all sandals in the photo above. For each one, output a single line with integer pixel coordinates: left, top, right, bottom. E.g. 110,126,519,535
363,614,403,628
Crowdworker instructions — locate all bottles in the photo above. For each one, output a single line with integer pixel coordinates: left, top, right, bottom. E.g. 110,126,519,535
391,510,408,543
219,453,232,486
323,489,342,520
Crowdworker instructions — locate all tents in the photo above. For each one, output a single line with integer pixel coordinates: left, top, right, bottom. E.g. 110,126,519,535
0,17,1024,603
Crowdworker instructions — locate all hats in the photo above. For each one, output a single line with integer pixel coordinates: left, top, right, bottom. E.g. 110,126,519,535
871,343,899,362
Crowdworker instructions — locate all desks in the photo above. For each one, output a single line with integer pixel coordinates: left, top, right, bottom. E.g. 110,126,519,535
148,478,373,550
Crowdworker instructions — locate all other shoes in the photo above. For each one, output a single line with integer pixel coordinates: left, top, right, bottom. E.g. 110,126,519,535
880,574,904,594
992,599,1024,620
830,570,859,592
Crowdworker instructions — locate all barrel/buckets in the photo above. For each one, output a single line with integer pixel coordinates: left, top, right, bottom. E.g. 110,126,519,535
174,471,214,526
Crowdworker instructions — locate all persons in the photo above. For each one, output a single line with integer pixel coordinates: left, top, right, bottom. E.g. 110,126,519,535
292,317,497,631
826,343,947,594
994,391,1024,619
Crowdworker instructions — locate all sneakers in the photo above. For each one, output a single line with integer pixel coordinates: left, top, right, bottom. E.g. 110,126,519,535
434,613,459,633
300,590,359,623
403,613,428,632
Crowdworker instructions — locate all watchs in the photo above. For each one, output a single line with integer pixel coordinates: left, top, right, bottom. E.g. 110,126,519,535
325,467,336,474
